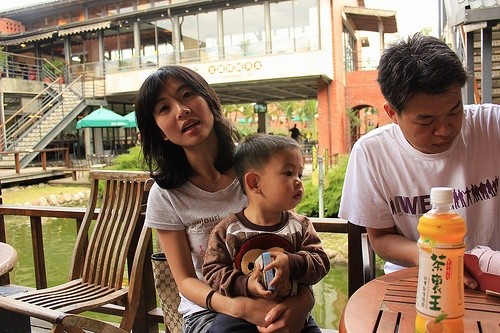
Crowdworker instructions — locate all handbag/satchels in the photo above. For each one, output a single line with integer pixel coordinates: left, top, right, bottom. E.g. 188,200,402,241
151,252,185,333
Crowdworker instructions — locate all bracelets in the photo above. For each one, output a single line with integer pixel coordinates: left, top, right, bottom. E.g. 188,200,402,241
206,288,216,312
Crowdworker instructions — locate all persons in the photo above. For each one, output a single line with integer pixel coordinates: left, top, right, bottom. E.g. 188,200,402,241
133,65,330,333
338,33,500,289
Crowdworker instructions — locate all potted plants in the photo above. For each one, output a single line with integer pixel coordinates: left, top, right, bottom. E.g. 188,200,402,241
43,64,51,82
0,47,6,77
54,59,65,82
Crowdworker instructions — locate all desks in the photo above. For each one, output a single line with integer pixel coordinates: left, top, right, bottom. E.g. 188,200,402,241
337,265,500,333
92,155,110,163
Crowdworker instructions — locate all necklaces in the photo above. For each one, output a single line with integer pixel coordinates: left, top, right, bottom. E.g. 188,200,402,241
202,170,220,192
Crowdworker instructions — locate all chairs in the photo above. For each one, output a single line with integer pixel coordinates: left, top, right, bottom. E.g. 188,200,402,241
0,169,154,333
76,153,104,179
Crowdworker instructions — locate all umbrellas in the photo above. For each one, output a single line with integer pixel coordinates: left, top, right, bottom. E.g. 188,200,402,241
77,105,139,155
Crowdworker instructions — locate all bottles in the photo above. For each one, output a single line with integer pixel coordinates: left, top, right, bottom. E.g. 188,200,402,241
414,187,468,333
